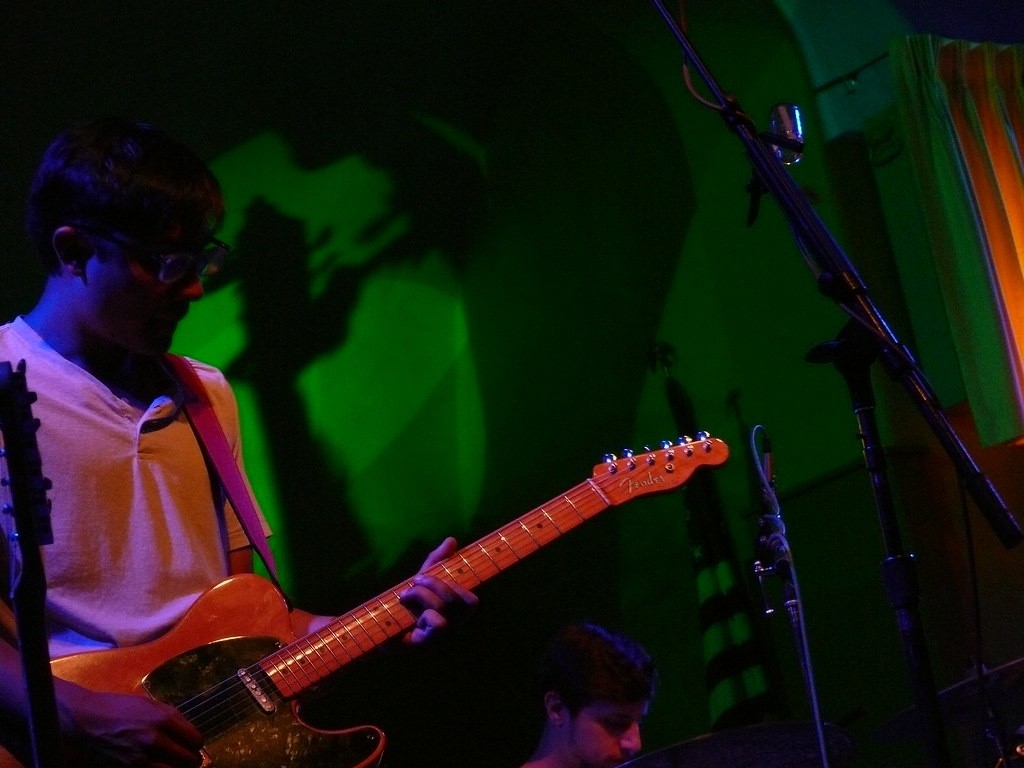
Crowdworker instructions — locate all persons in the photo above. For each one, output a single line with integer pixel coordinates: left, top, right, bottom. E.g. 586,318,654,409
1,118,480,768
515,624,656,768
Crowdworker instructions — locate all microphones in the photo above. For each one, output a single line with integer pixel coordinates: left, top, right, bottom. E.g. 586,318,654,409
760,435,775,501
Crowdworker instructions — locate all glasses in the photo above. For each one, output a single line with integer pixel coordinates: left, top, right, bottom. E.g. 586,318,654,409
73,225,228,299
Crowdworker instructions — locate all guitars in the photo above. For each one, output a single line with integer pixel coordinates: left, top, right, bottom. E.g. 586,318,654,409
0,424,732,768
